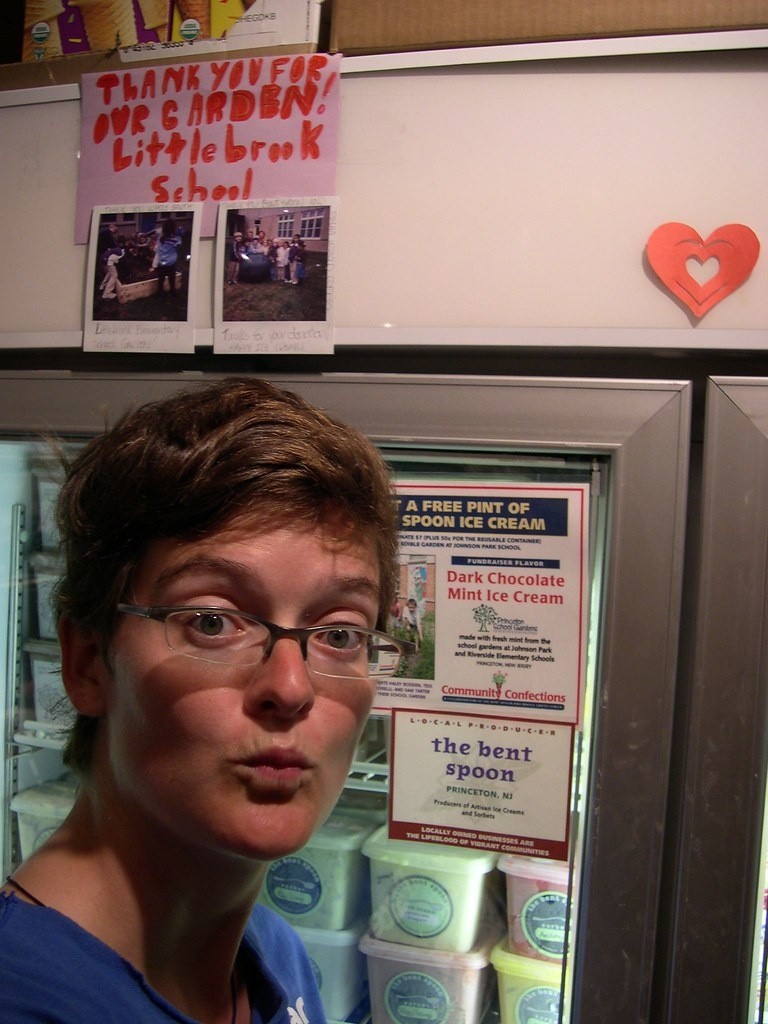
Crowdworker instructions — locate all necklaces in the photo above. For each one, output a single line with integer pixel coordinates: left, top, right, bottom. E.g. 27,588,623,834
6,870,238,1024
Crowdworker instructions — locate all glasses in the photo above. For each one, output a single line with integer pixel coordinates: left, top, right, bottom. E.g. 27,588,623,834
111,605,417,681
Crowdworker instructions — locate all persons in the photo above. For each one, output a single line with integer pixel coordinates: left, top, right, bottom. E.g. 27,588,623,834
118,229,156,260
229,232,242,286
97,224,118,265
402,599,424,651
100,248,132,298
242,229,306,285
0,375,414,1023
155,219,181,295
388,597,403,636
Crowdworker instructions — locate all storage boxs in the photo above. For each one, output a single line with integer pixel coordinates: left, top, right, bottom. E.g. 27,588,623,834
254,822,572,1024
22,456,81,735
12,773,89,871
